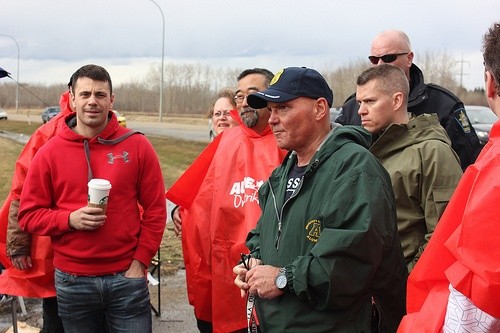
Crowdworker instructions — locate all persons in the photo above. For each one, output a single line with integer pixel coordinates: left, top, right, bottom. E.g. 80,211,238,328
334,28,483,174
395,25,500,332
165,66,287,332
18,65,166,333
232,67,409,333
165,90,255,333
6,75,79,333
354,64,465,275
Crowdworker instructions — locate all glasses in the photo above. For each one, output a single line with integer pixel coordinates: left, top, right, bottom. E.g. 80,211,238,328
233,94,250,103
240,252,257,271
212,109,231,117
367,53,410,64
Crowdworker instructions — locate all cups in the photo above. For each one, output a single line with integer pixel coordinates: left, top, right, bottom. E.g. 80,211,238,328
87,179,112,215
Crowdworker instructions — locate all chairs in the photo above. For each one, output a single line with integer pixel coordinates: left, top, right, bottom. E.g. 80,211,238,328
0,243,161,333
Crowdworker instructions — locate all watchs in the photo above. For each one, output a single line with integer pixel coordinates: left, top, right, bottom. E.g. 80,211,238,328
274,267,290,293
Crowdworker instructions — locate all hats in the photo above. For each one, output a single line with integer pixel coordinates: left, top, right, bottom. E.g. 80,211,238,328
247,66,333,109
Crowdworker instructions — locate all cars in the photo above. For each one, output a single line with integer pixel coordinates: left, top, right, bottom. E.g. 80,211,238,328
463,105,499,147
41,106,61,124
0,110,8,119
111,110,127,129
